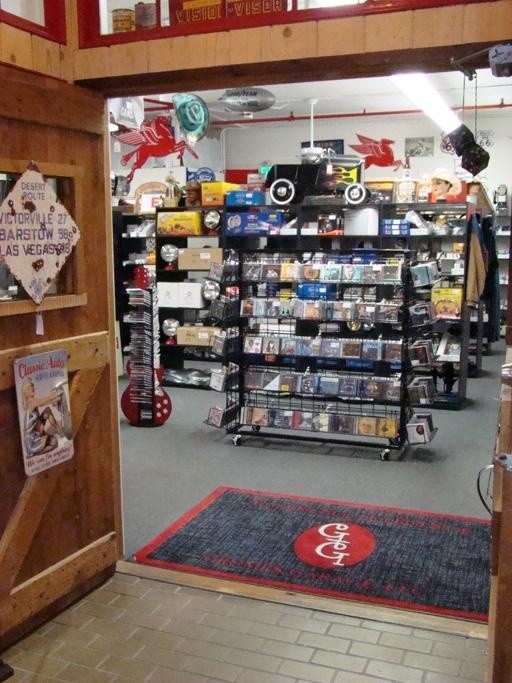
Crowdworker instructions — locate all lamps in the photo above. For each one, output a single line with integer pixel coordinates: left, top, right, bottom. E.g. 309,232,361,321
446,72,490,175
301,98,324,164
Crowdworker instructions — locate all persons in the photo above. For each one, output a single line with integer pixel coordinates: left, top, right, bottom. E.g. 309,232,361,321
423,167,462,205
23,377,64,456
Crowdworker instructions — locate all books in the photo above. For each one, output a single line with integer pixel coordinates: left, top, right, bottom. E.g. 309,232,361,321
123,286,161,404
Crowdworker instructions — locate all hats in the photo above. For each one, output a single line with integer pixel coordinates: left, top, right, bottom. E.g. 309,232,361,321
172,93,209,142
432,169,463,195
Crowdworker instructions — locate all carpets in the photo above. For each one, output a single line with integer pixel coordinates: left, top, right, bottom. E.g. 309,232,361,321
131,487,493,623
